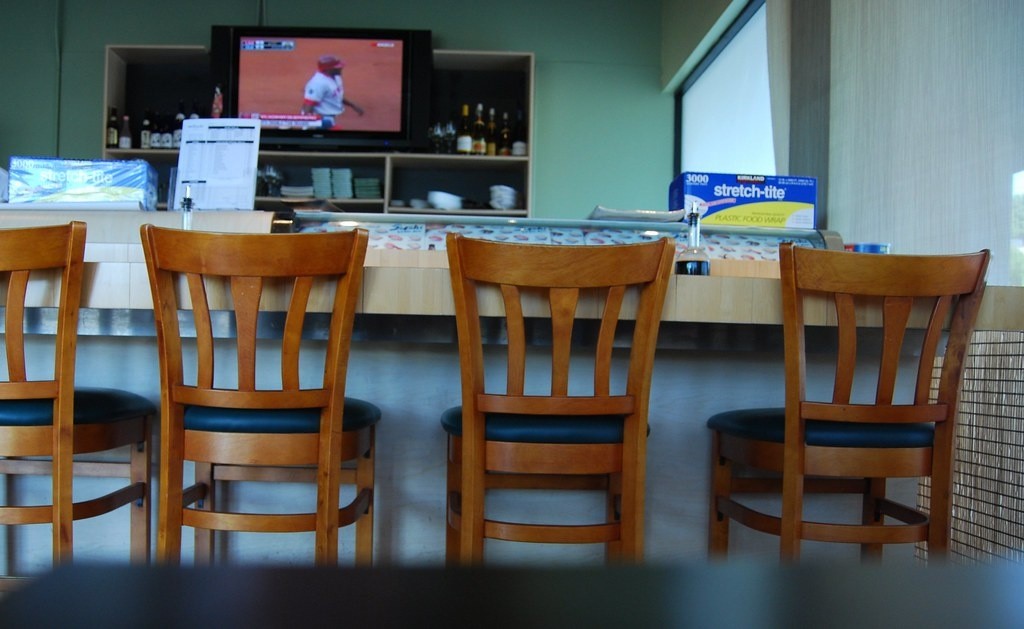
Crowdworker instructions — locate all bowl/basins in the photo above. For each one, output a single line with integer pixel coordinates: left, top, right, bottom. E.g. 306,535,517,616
490,183,516,209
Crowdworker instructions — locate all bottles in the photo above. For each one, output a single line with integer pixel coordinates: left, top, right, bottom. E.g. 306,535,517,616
152,112,163,148
457,105,472,153
119,115,131,148
173,97,186,147
140,107,152,149
163,111,173,149
188,100,200,120
106,104,119,148
473,103,487,154
488,105,497,156
499,112,511,155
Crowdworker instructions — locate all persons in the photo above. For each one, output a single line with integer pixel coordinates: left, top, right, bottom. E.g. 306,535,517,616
301,56,366,128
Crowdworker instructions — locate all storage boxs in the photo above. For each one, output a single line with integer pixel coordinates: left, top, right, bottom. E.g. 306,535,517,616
8,155,160,212
669,171,818,229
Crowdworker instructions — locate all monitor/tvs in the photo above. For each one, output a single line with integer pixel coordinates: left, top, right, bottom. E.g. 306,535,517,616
210,24,433,149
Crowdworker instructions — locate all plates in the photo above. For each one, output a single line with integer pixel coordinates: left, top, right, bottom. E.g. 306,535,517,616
280,167,381,199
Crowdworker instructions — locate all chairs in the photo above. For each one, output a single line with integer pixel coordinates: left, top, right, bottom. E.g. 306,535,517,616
141,223,383,569
706,243,992,565
0,220,157,592
440,232,678,569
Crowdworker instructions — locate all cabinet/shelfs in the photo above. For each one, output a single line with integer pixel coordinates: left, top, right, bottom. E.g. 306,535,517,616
101,44,535,219
